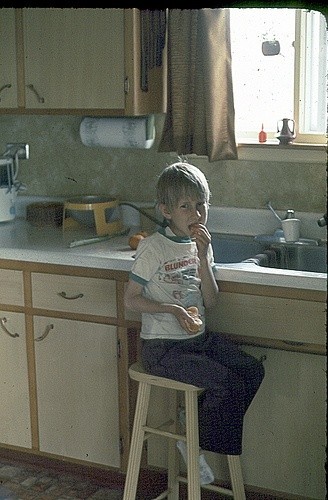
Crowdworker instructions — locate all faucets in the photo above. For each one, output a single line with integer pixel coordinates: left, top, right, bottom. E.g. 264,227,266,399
254,200,322,245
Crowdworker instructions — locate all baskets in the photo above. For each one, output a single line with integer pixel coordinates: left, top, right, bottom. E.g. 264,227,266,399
25,200,63,226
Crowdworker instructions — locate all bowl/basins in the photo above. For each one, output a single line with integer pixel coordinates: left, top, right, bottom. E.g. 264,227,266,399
26,201,63,228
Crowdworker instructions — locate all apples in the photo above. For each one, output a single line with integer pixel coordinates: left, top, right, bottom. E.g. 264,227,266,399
129,230,149,250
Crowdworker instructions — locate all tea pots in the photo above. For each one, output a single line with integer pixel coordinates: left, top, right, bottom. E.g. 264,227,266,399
275,118,296,144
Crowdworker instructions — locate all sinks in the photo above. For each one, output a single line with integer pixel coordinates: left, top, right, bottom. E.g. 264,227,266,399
132,234,276,284
247,243,328,292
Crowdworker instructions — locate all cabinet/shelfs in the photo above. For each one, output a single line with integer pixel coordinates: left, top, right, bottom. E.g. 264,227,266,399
0,8,171,119
0,257,328,500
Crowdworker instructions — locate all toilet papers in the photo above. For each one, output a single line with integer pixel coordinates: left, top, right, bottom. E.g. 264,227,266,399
79,116,155,150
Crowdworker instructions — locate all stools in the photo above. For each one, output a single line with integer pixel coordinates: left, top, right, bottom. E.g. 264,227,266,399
122,358,249,500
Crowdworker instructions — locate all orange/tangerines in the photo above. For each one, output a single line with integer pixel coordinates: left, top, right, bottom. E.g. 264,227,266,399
185,306,202,330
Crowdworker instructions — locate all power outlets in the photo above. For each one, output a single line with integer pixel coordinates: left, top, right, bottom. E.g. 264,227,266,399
6,142,30,161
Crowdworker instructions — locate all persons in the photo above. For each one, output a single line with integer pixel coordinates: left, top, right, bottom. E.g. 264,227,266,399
123,162,264,485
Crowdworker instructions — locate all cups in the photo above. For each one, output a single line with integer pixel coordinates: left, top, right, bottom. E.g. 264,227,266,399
281,218,300,242
